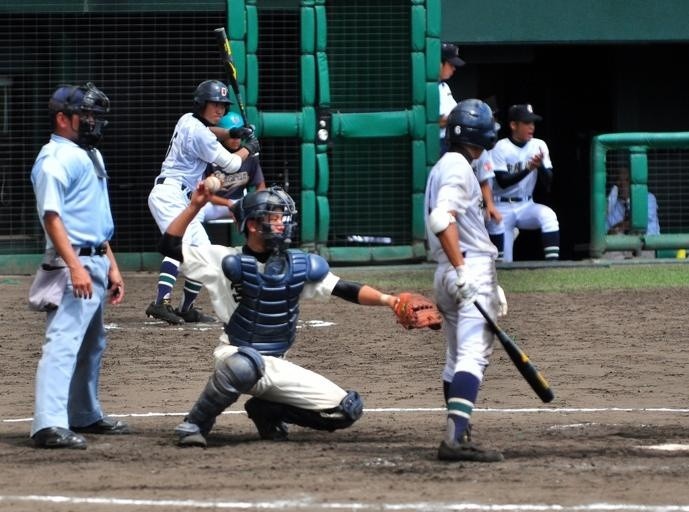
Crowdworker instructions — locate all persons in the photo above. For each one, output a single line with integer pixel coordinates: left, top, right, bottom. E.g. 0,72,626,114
422,96,509,465
160,177,399,449
439,40,459,154
145,79,260,325
201,110,267,223
27,83,131,449
607,163,662,235
472,148,503,223
486,101,562,261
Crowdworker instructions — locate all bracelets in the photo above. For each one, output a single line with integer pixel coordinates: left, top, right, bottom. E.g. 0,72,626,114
380,291,393,308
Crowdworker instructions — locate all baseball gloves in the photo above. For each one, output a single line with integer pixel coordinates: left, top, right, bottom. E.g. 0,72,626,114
391,292,444,331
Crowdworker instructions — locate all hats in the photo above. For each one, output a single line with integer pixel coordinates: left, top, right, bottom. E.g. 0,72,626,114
507,104,541,124
48,82,107,116
441,42,466,67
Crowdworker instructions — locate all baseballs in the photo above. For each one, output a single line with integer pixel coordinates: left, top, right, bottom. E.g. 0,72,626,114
204,176,221,192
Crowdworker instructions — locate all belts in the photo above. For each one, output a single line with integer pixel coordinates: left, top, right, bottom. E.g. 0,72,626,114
156,178,193,202
500,195,534,202
78,246,107,257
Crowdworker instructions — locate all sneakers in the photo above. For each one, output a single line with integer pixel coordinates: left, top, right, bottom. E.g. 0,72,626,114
437,442,507,463
145,300,185,325
242,396,288,441
175,304,219,325
176,429,209,447
33,426,87,450
68,417,127,435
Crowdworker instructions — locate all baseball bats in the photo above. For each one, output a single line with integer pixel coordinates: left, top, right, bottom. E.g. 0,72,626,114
215,29,249,128
472,298,555,403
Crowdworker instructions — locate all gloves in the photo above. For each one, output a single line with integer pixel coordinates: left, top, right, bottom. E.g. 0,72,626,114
243,137,261,155
497,285,508,317
455,266,479,303
230,121,254,138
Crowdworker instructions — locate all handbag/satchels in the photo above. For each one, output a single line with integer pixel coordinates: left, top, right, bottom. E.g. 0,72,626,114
25,262,69,313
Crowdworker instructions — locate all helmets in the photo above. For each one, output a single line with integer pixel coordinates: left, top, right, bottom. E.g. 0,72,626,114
216,110,245,135
195,79,232,116
234,186,298,233
446,97,501,151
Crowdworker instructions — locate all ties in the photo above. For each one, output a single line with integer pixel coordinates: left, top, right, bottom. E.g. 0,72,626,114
624,201,631,235
